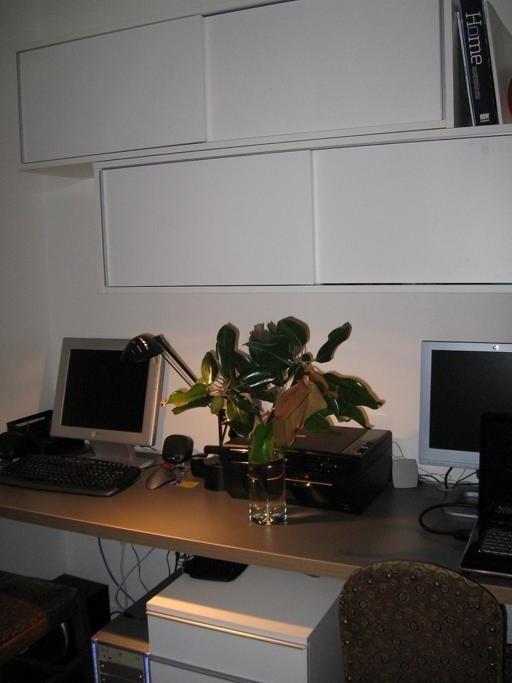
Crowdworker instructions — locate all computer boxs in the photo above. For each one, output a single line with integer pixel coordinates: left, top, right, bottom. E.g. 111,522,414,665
89,569,185,682
52,573,111,663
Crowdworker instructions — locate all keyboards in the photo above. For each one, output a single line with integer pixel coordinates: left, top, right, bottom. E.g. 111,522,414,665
0,452,142,497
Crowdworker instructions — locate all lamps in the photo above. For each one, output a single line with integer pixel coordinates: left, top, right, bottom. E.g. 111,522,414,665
119,332,230,492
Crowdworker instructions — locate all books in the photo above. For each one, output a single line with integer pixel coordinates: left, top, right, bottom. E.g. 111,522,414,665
455,0,510,124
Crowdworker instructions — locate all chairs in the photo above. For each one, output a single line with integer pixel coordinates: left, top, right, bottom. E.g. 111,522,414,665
339,556,510,683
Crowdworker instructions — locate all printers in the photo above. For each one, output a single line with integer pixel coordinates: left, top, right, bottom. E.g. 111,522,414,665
219,428,392,511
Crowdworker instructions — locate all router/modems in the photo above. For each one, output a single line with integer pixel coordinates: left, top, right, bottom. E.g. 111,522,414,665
185,556,248,582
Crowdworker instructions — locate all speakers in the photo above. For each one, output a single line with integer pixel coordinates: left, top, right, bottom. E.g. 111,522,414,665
162,434,193,464
390,459,418,489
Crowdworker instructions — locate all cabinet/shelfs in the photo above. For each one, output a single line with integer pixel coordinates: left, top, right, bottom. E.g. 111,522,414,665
315,126,512,294
90,145,316,295
140,561,346,683
205,0,512,153
11,13,209,169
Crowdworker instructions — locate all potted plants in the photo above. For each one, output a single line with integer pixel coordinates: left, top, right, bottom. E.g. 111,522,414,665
155,312,386,526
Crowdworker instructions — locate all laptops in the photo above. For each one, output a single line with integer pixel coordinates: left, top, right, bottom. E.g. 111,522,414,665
458,411,512,578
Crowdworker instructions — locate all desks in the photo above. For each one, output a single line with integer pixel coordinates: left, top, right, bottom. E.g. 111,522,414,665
0,451,512,608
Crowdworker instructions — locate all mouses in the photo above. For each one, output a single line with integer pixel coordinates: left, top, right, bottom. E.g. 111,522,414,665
147,466,174,489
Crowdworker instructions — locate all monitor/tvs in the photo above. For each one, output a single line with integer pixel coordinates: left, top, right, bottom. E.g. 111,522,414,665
49,337,164,466
419,338,512,518
